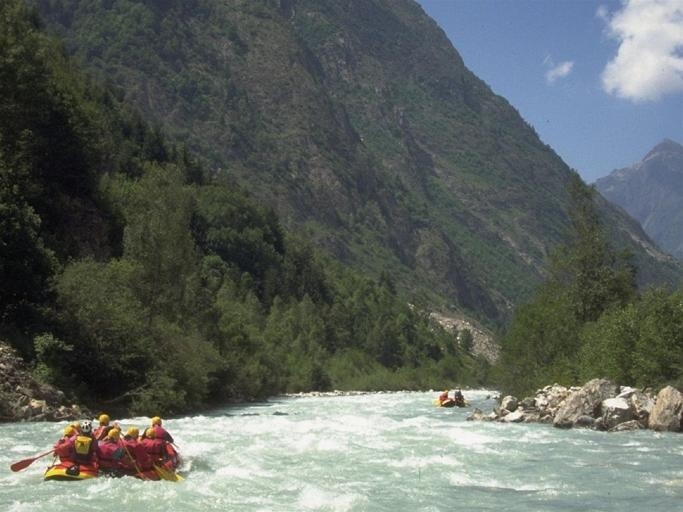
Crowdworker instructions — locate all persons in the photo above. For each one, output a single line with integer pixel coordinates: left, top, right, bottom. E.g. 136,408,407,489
53,414,176,473
455,387,463,399
440,390,449,401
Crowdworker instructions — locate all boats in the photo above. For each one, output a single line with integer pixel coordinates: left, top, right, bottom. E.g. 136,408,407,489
432,398,466,407
43,435,180,480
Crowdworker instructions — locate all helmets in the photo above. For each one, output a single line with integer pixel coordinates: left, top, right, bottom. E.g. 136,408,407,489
64,414,162,442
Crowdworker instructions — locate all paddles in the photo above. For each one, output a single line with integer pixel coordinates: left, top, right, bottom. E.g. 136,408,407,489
152,461,183,481
119,437,151,481
11,449,55,472
